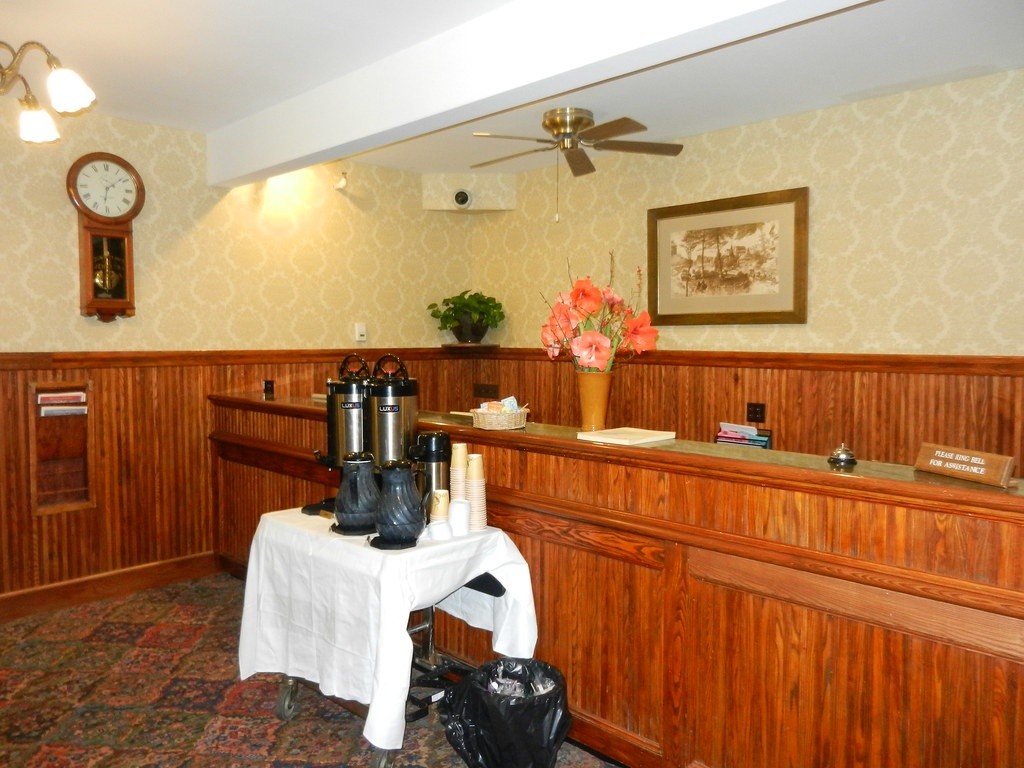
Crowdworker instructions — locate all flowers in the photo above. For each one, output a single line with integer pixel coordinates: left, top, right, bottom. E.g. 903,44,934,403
537,250,659,372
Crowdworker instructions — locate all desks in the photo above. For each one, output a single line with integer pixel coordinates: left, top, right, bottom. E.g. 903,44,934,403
239,503,538,768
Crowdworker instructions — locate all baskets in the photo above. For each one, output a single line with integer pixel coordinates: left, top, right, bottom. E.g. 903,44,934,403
470,403,530,431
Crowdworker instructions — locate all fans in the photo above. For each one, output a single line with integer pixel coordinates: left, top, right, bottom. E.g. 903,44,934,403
471,108,684,223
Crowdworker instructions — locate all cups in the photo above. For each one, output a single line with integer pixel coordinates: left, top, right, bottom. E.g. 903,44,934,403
448,443,468,500
430,489,449,523
427,522,453,542
466,453,488,534
450,499,471,539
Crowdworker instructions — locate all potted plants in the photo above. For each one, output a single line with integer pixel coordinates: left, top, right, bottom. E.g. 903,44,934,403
426,289,505,344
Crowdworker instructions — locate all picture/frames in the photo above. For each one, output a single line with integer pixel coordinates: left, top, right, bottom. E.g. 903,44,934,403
647,186,808,324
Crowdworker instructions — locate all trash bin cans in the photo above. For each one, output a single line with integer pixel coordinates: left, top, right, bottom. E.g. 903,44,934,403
469,657,568,768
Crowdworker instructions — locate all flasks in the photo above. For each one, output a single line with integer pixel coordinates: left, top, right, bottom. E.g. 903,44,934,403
409,430,450,521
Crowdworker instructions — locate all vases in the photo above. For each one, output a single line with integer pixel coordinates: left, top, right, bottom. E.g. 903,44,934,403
575,371,613,432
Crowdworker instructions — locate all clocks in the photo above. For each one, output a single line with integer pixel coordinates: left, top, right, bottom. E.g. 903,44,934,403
66,152,145,322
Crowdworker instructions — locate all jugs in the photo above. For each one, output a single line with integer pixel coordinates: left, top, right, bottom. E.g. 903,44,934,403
375,460,433,542
333,451,382,530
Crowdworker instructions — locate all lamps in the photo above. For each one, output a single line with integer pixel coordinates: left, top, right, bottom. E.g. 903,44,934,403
0,39,96,144
